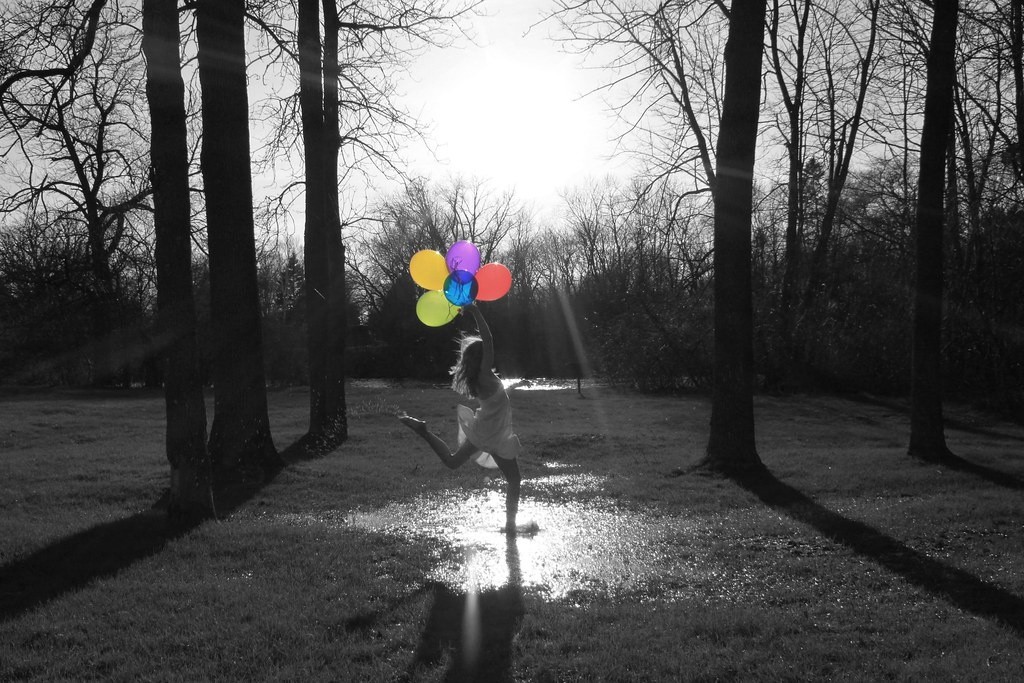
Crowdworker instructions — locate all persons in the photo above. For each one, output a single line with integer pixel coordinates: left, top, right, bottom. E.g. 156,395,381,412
397,304,533,532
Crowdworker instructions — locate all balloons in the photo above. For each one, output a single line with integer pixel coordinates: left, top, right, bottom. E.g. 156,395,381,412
409,240,512,327
